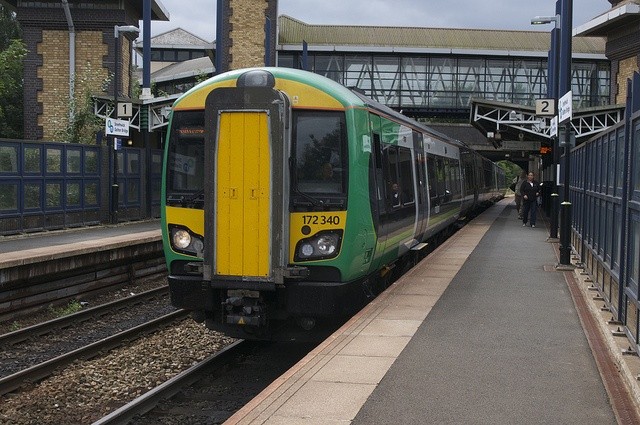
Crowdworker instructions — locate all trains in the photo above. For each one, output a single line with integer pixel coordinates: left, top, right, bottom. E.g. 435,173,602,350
161,66,507,345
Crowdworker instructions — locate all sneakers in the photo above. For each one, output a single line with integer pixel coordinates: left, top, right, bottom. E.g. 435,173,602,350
522,223,526,227
530,224,535,228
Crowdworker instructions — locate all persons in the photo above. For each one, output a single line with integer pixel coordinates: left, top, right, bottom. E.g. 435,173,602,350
520,170,544,228
509,169,527,221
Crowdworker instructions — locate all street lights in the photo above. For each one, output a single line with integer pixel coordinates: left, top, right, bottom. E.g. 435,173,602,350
531,15,560,238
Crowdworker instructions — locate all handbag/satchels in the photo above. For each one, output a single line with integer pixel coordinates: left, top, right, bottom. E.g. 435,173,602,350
510,175,519,192
